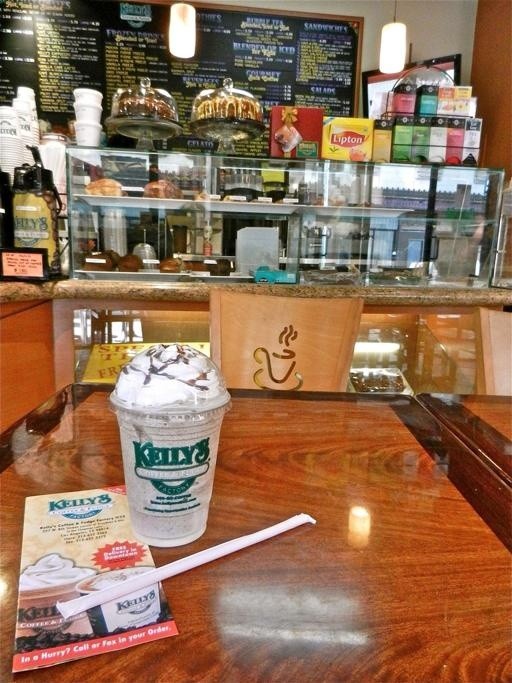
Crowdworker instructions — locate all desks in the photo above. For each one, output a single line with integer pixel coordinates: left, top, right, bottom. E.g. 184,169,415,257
2,382,512,683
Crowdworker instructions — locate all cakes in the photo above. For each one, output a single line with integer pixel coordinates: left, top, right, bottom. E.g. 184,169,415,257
115,95,176,118
194,95,263,118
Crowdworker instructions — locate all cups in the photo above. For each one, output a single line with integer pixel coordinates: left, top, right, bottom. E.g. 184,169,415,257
108,344,235,549
1,87,40,186
71,88,103,146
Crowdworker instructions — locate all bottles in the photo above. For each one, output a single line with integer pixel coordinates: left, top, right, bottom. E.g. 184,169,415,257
0,133,69,277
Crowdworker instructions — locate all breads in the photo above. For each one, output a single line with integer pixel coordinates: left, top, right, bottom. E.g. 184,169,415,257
144,179,185,199
159,256,181,273
211,259,233,275
84,250,145,273
86,177,124,196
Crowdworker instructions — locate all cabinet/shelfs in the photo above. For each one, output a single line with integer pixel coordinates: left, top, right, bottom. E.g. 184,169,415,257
64,147,505,283
